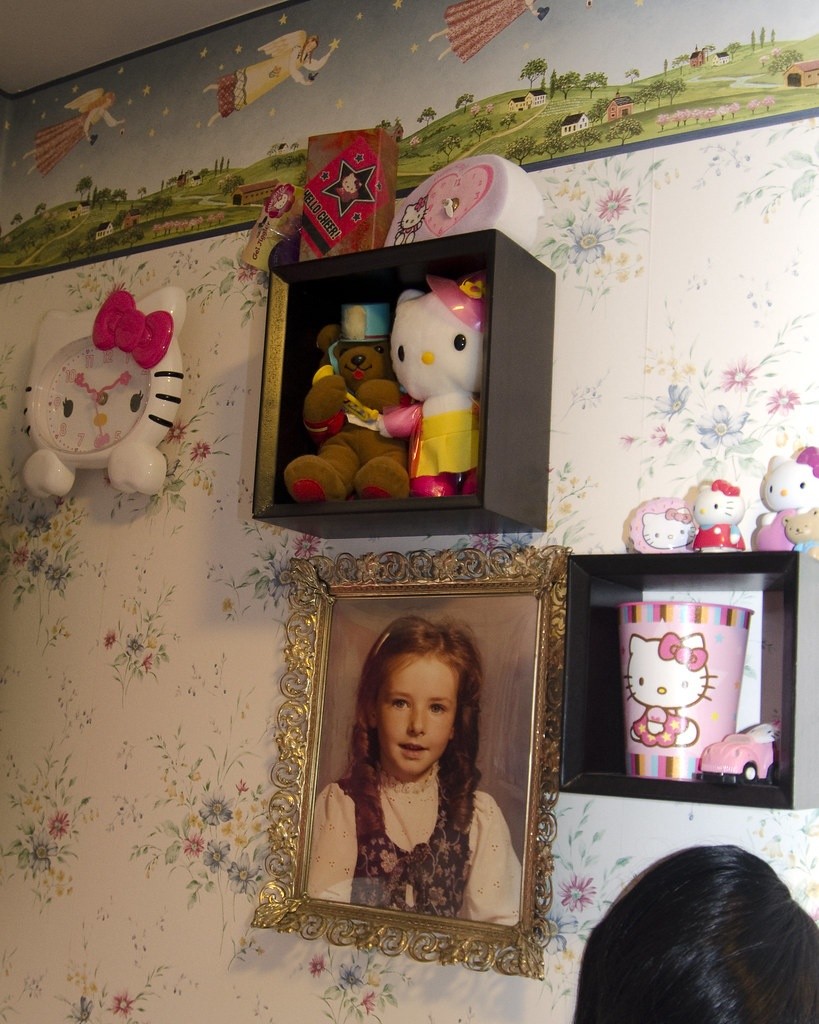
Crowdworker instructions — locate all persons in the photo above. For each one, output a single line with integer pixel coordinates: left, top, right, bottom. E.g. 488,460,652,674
572,847,819,1024
306,615,522,926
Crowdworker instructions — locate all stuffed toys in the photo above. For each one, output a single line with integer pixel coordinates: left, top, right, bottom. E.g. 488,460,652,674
376,274,484,497
284,303,410,503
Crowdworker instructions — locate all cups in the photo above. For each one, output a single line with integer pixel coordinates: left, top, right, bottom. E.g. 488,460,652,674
615,599,757,782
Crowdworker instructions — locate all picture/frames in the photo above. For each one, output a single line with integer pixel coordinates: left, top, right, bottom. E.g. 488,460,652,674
249,545,572,980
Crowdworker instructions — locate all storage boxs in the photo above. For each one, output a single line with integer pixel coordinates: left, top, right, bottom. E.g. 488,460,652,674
556,549,819,811
247,226,556,538
296,127,400,258
